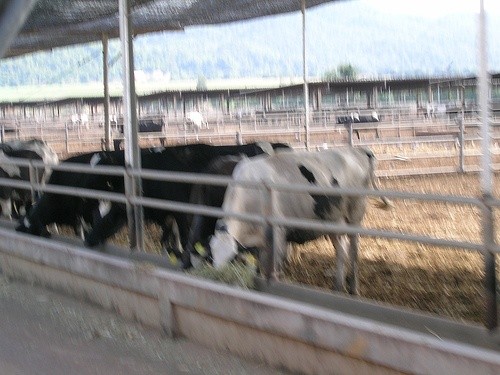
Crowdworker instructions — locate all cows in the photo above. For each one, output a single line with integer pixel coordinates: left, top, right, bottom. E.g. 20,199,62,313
205,147,393,296
84,143,295,262
0,138,58,233
116,116,166,146
185,111,210,141
68,114,89,131
17,151,124,235
337,114,380,138
